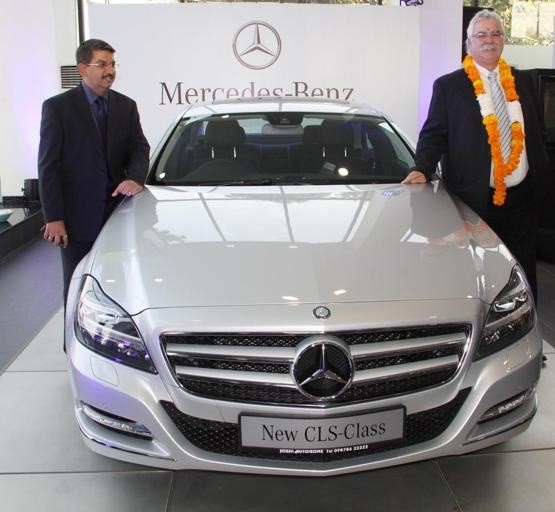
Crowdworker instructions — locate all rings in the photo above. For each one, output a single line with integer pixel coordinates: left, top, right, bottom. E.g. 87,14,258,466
63,234,68,238
48,237,51,240
127,191,131,195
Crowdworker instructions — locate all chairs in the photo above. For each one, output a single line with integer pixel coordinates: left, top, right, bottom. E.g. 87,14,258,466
183,117,269,183
298,119,364,182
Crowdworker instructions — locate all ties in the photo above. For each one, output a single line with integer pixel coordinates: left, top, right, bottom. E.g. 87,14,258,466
488,72,513,165
95,96,107,135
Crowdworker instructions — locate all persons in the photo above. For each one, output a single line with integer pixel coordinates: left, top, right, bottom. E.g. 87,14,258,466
401,6,548,364
36,39,152,356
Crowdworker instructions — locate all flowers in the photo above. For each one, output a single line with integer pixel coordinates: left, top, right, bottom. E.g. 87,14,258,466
463,55,526,207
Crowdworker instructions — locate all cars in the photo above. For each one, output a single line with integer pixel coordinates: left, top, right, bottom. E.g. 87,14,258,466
65,97,543,476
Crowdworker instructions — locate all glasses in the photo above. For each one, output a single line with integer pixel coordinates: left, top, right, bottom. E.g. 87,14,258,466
86,61,120,69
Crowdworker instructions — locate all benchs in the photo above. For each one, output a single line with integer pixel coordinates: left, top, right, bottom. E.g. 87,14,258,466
246,142,319,175
